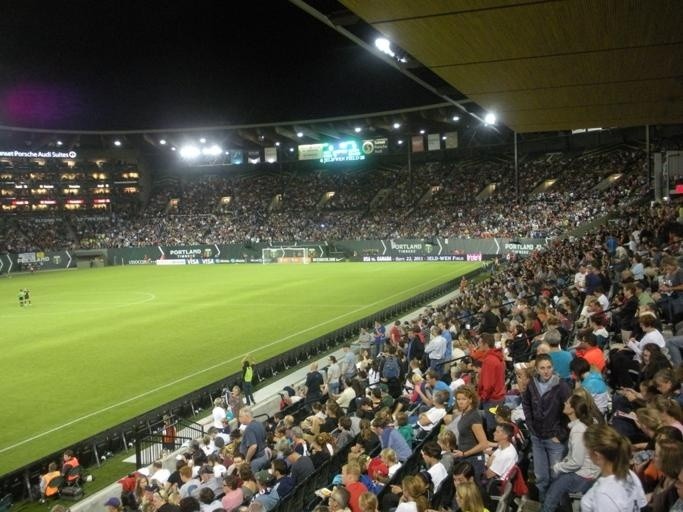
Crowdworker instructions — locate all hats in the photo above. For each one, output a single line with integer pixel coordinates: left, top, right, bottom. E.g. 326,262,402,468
489,404,511,418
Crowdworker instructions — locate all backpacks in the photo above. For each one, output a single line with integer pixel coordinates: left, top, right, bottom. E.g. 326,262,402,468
382,355,401,378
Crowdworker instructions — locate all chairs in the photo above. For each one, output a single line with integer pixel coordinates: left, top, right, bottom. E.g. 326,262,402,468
39,476,65,505
231,377,582,512
63,465,82,487
0,492,14,512
